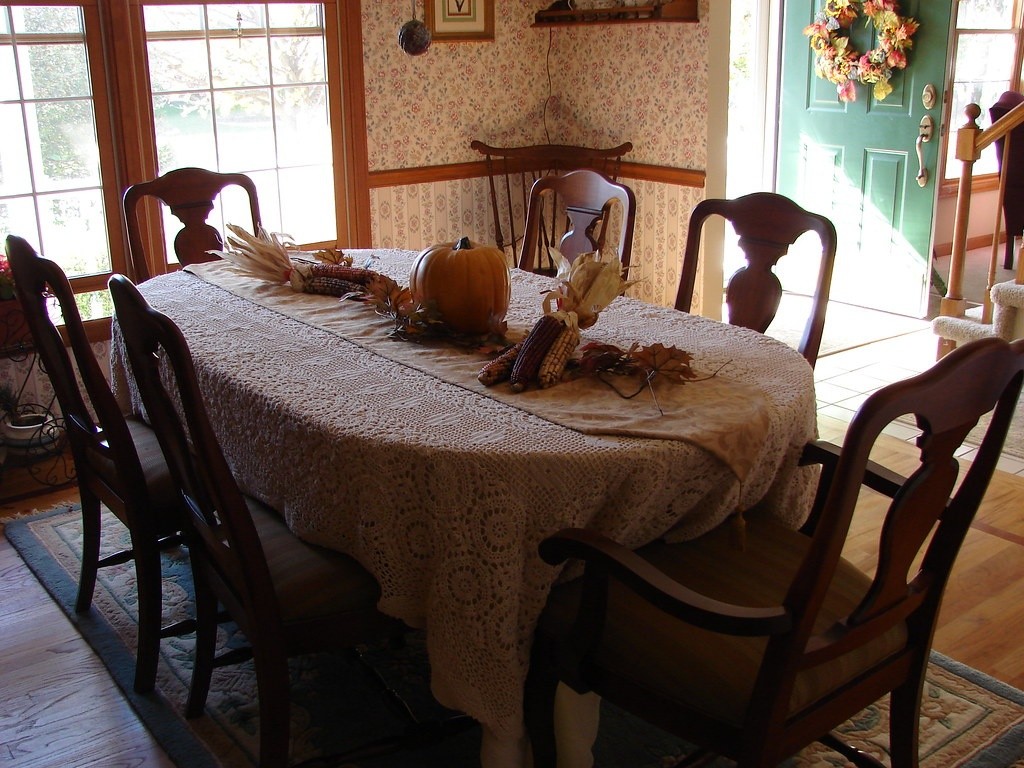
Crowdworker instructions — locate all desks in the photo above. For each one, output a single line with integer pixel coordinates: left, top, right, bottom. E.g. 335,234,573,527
109,245,824,768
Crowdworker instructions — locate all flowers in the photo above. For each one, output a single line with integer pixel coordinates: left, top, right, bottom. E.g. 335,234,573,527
804,0,919,103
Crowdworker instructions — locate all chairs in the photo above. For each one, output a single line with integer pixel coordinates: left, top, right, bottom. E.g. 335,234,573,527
122,167,262,286
105,272,428,768
520,168,637,297
523,340,1024,768
674,192,839,371
471,140,633,278
15,239,200,695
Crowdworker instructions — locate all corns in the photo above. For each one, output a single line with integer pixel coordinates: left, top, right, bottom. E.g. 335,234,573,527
477,314,579,393
304,264,381,296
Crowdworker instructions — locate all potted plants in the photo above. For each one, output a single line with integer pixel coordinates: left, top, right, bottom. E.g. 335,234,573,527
0,254,15,301
0,385,59,456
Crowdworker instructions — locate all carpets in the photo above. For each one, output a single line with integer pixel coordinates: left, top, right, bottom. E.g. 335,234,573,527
2,498,1024,768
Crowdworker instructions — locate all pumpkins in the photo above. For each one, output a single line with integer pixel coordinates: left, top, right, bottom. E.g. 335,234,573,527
409,236,511,334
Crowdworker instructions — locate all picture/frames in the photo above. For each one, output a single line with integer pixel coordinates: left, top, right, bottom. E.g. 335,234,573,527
423,0,496,43
